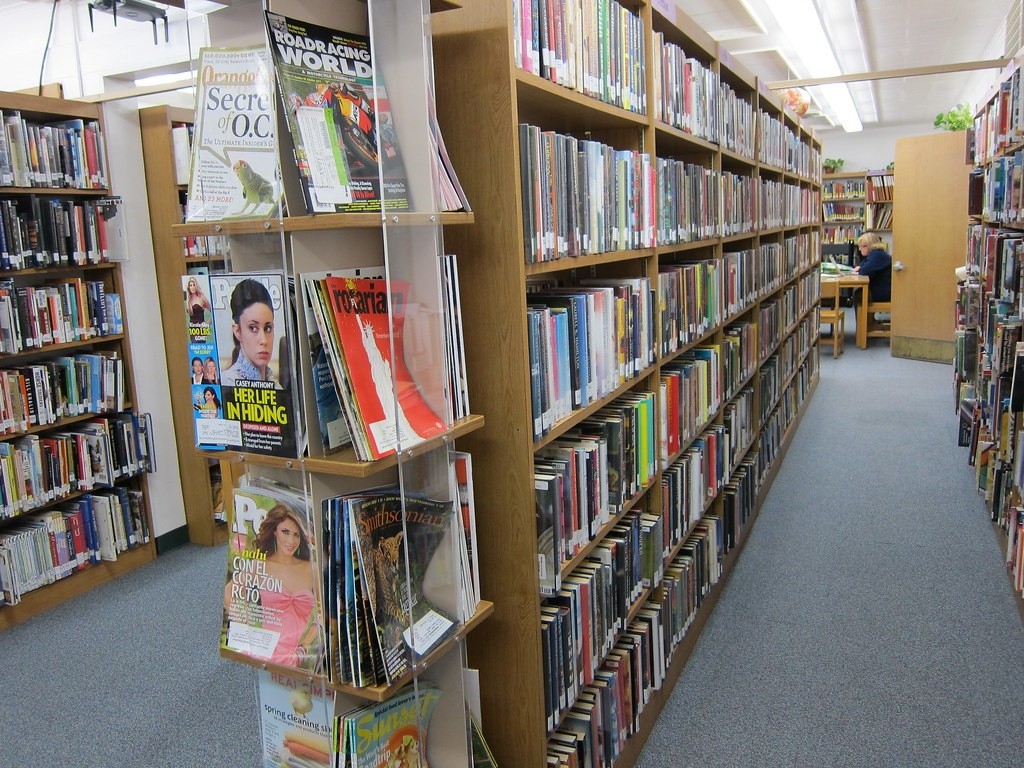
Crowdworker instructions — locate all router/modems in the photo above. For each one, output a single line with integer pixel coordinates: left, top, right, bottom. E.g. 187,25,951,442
88,0,168,45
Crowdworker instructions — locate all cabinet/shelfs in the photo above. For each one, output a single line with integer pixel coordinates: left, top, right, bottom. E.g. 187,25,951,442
0,1,1024,768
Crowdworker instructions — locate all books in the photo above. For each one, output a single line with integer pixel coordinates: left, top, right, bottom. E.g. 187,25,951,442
170,3,820,768
0,109,157,606
821,176,893,244
951,70,1024,598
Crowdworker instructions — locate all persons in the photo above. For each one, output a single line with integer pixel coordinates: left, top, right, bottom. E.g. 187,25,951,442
854,232,892,332
